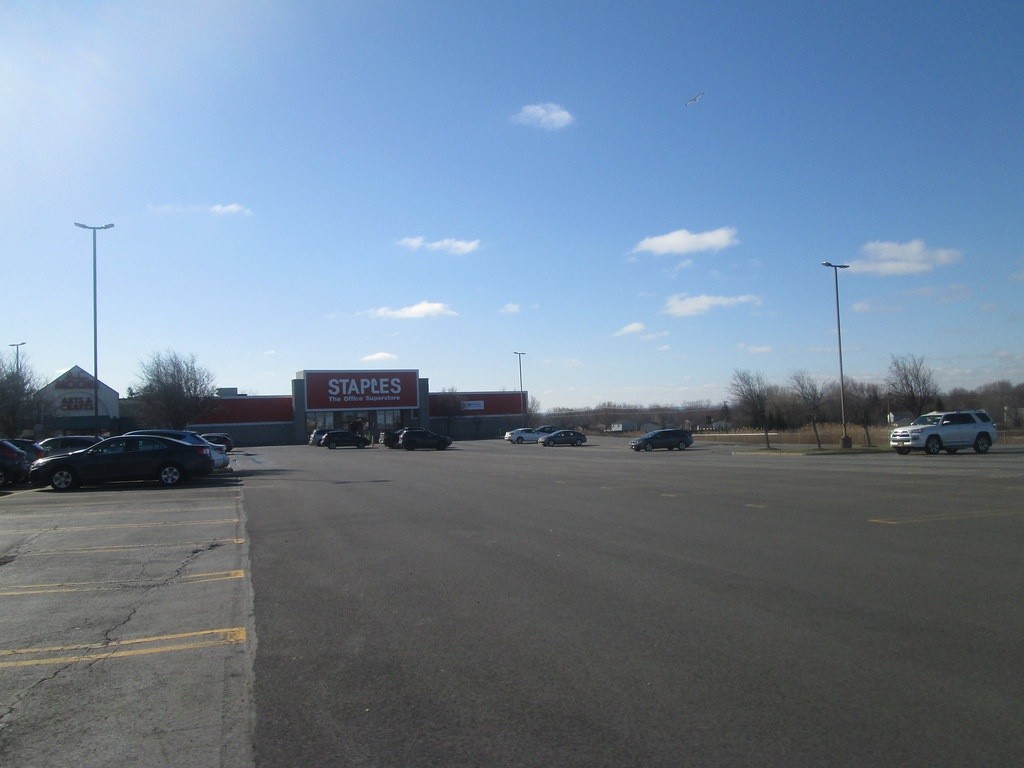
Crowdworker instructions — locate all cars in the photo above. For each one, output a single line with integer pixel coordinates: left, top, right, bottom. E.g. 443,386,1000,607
309,428,336,447
504,427,551,444
537,429,587,447
378,426,426,449
320,431,371,449
628,428,695,452
534,424,562,435
0,428,235,492
27,433,215,493
396,427,452,450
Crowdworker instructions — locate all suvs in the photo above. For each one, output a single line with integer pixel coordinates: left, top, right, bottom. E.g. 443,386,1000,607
889,407,998,456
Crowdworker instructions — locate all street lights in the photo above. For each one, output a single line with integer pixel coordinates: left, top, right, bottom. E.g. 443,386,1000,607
8,341,28,376
820,260,854,449
75,222,115,445
514,351,525,428
